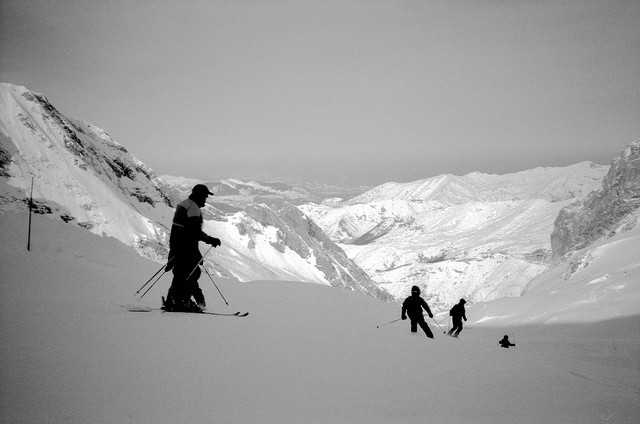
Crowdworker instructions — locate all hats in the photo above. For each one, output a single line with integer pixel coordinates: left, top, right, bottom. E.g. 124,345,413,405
192,184,214,196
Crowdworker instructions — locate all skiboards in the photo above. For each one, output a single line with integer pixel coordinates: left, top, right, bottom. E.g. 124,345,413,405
121,298,249,317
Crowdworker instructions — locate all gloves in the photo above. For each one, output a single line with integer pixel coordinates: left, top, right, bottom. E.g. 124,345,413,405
206,236,220,248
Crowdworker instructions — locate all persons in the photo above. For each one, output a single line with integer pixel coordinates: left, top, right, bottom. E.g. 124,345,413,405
447,298,467,337
166,184,215,312
165,249,206,308
400,286,435,339
499,335,516,348
